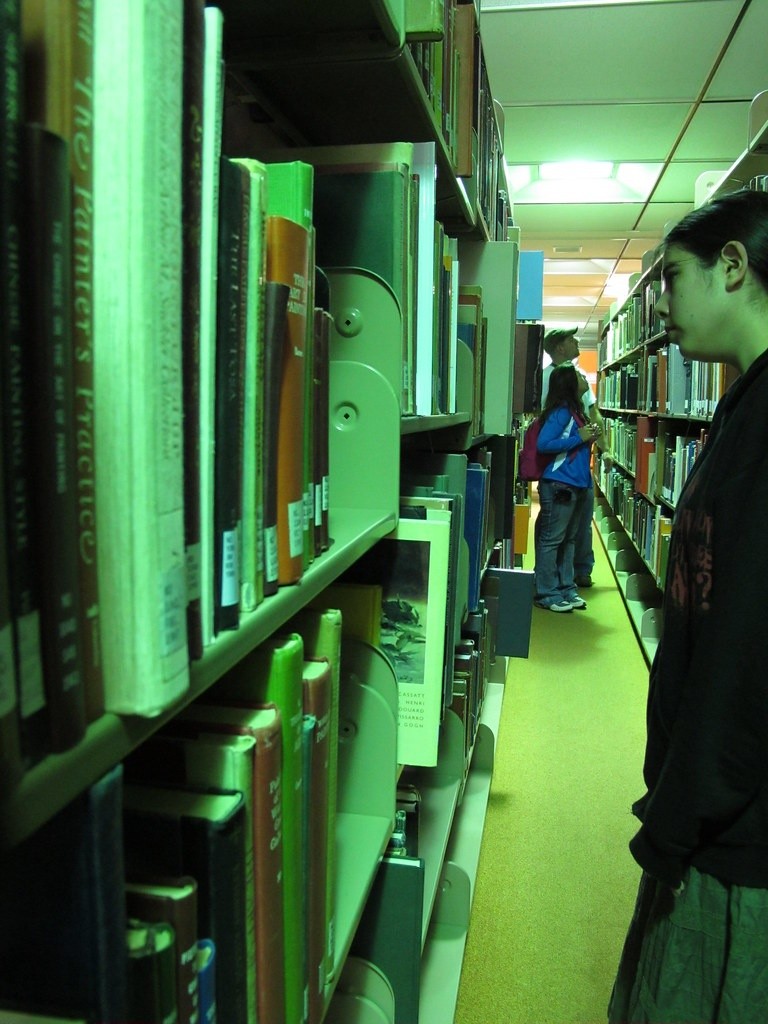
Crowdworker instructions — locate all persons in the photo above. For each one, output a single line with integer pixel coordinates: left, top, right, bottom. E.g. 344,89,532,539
605,189,768,1024
533,363,602,611
540,327,606,589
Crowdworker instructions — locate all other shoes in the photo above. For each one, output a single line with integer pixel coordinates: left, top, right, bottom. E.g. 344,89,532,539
574,574,592,588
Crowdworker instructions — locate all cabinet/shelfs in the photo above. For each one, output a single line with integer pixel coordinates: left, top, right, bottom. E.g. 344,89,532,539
2,0,768,1024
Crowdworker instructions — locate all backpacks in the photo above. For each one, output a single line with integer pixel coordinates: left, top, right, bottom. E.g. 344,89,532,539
519,407,587,481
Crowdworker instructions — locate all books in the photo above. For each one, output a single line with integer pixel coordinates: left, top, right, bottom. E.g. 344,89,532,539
597,176,723,593
0,1,513,1023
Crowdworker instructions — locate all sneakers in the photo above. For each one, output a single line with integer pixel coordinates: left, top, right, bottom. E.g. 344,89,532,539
568,595,586,608
536,600,574,612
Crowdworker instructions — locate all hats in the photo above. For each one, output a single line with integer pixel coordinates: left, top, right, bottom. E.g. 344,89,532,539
543,327,577,354
